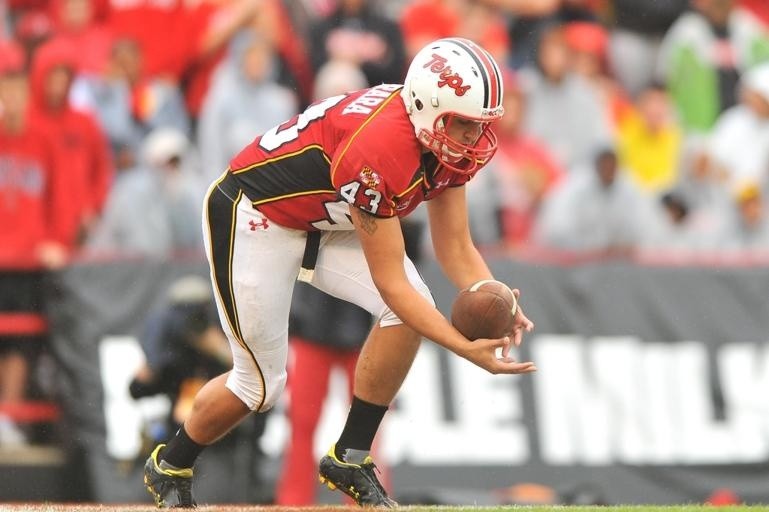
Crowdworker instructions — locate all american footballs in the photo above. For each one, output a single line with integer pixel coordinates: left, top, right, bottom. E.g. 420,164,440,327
451,280,517,341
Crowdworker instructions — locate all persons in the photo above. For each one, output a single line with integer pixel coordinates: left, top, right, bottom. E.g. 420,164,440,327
0,0,767,274
129,274,279,505
139,35,538,512
1,67,62,447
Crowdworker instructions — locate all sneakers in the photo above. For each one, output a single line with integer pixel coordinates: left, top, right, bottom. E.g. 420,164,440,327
144,444,197,508
318,446,398,509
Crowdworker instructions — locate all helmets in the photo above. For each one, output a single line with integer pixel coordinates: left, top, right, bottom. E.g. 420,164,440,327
400,37,505,176
